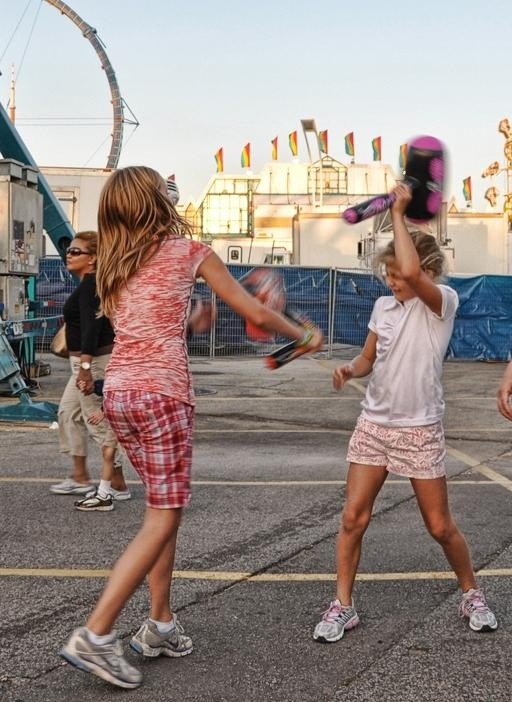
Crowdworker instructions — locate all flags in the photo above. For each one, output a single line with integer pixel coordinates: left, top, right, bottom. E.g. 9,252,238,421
344,132,354,156
371,136,381,161
462,176,472,202
241,143,250,168
288,130,298,156
398,143,407,168
271,137,278,160
319,130,328,154
214,147,224,173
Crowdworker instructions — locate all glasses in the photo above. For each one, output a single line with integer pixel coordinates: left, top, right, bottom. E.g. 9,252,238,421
66,247,90,255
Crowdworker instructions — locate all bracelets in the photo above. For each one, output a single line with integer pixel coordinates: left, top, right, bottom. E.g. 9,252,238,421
295,326,313,346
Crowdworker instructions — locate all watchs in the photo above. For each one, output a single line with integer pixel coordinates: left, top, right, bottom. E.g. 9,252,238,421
79,362,91,370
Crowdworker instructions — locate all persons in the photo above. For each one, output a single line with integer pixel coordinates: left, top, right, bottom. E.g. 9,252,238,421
47,228,132,500
311,181,500,642
73,379,119,511
496,360,512,422
57,165,323,692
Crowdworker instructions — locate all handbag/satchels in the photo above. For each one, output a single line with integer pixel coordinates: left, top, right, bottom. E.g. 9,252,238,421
49,323,68,359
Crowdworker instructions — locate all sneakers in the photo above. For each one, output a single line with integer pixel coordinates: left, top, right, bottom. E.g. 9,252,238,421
74,491,114,512
457,588,498,632
85,487,131,501
129,613,193,657
59,626,143,689
314,597,359,643
49,478,94,497
